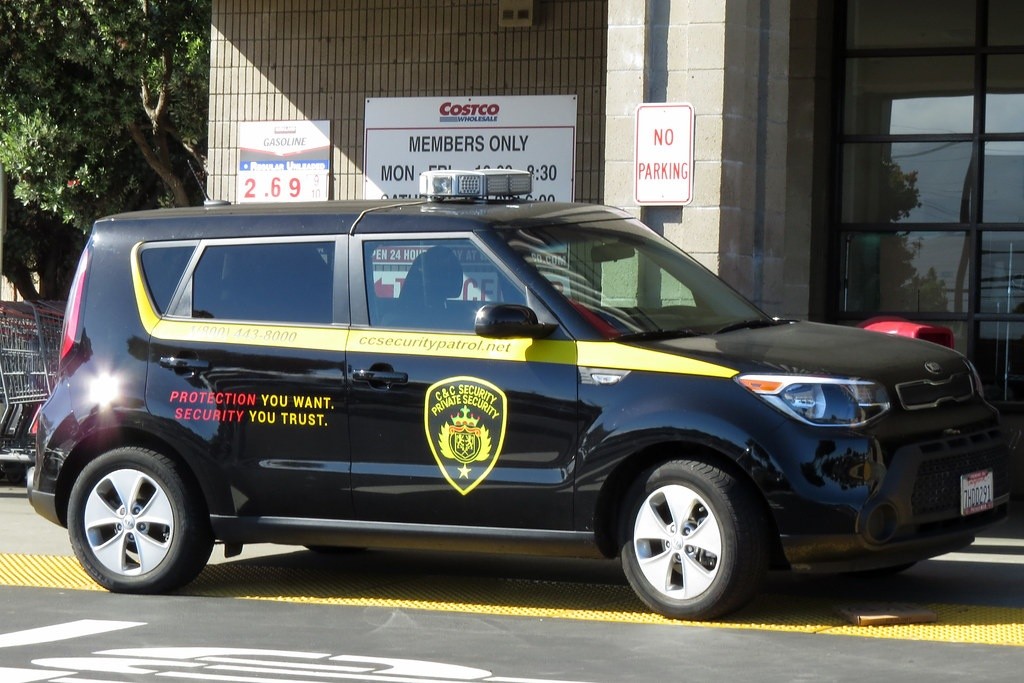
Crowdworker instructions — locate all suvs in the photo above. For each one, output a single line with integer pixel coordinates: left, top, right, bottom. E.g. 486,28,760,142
30,170,1015,621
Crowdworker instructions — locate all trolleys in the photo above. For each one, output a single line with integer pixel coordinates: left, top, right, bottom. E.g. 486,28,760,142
0,299,64,483
